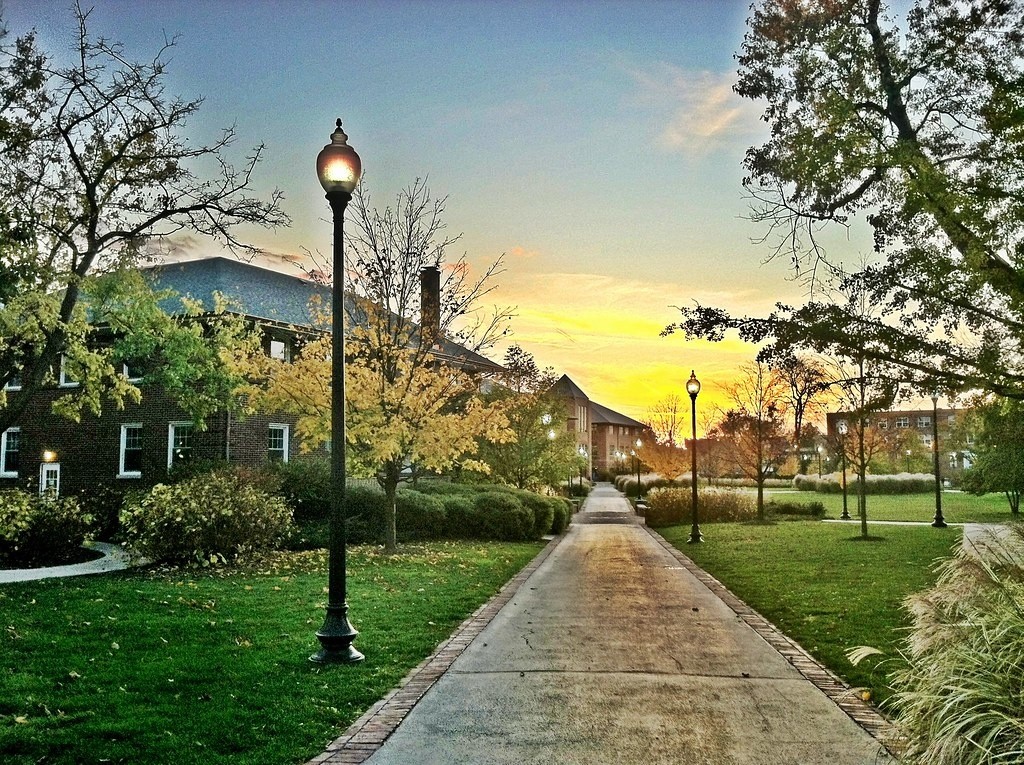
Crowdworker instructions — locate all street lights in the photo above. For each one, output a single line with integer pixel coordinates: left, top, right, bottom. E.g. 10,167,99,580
621,453,626,474
578,448,584,495
930,391,948,526
615,452,620,476
818,446,823,478
548,430,555,497
906,449,911,471
631,450,635,477
309,118,366,667
686,369,704,543
839,422,850,518
636,438,642,500
